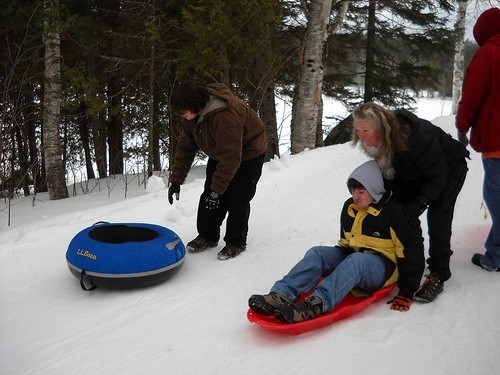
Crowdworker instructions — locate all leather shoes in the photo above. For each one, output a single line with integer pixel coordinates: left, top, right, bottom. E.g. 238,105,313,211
471,252,500,272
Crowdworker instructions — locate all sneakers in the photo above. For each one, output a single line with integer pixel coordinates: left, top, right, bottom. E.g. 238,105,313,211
414,276,444,302
249,293,292,320
280,294,323,324
217,243,246,260
186,235,220,252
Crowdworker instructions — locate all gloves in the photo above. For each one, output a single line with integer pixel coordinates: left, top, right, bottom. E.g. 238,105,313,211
387,295,412,312
168,183,181,204
205,192,222,210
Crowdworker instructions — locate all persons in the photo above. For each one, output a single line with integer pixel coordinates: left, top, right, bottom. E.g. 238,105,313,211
247,160,421,323
456,7,500,272
350,102,472,305
167,78,268,260
